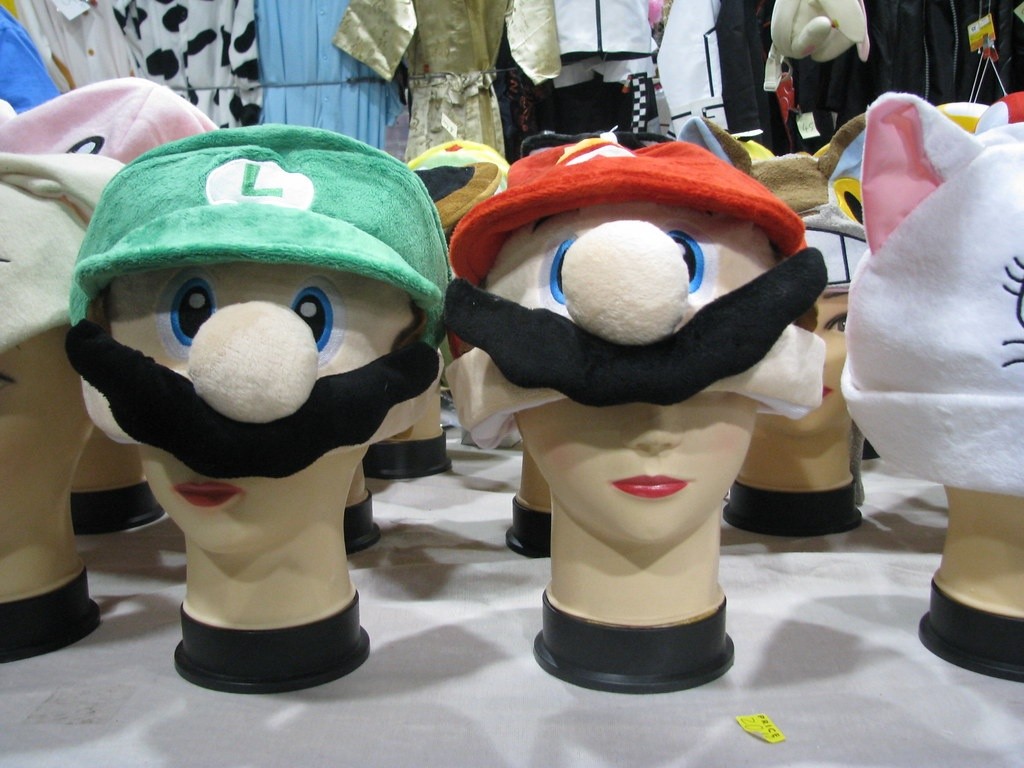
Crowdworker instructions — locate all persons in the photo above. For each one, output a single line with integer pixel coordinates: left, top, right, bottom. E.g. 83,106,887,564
444,137,826,696
0,76,1024,552
838,92,1024,683
67,125,451,694
0,153,127,665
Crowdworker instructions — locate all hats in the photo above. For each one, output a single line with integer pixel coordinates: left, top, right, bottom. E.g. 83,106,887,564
0,77,1024,498
